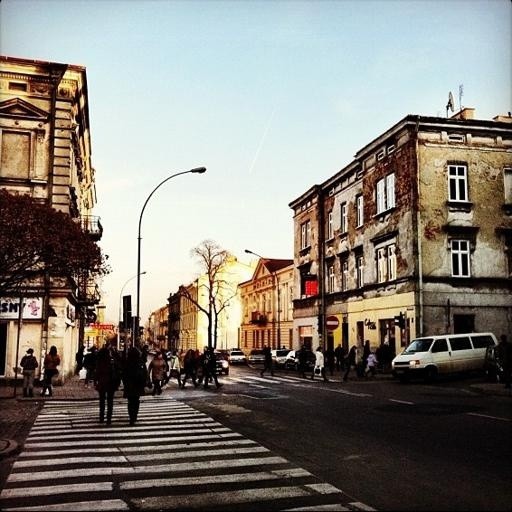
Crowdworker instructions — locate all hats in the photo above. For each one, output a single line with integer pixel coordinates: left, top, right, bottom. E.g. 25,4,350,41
26,349,33,352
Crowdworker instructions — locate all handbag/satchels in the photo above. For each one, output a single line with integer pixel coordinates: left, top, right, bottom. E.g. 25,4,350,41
170,369,180,378
45,369,58,376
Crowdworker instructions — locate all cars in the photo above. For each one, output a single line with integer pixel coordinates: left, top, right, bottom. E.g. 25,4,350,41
214,351,229,376
271,348,316,373
227,351,247,366
248,349,266,364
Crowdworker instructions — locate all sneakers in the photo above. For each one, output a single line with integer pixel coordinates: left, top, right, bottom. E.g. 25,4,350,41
152,390,161,395
179,380,224,389
100,418,111,424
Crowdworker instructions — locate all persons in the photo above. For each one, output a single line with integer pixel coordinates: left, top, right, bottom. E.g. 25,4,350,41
299,340,379,383
75,343,224,426
261,346,275,377
20,348,38,397
40,346,60,397
489,334,512,388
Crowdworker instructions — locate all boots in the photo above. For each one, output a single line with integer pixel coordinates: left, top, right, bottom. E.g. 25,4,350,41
23,385,53,397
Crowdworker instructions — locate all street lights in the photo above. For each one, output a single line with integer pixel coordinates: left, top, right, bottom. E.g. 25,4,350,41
118,272,146,320
135,168,206,348
245,250,281,351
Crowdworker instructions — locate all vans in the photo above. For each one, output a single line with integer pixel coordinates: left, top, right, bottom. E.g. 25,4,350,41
390,332,499,382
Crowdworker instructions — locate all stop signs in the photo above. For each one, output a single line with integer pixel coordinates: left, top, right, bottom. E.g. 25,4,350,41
326,316,339,331
